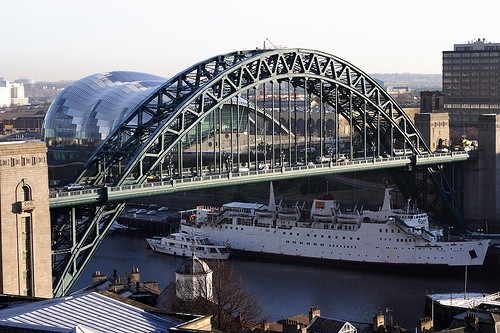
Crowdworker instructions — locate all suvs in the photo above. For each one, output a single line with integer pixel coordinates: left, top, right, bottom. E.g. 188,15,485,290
148,204,158,210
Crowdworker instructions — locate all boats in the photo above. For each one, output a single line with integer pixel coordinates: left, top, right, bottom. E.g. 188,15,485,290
145,225,233,261
169,179,494,270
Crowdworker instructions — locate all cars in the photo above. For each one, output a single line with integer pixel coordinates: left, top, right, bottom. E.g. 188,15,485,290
135,208,147,215
124,139,478,183
62,182,85,191
146,209,158,216
126,208,138,213
158,206,169,212
